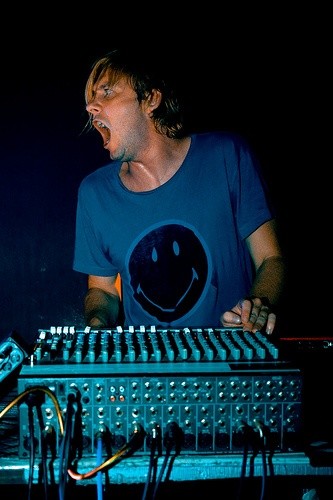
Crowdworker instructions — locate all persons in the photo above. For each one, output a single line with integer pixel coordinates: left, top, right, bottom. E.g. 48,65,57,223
68,47,287,337
